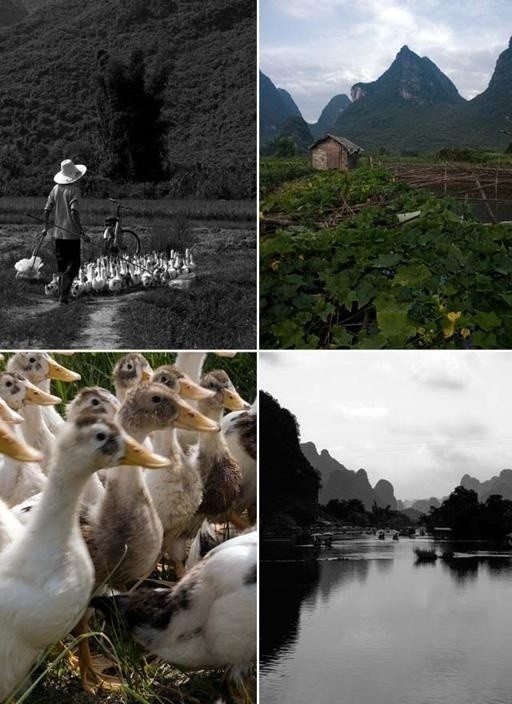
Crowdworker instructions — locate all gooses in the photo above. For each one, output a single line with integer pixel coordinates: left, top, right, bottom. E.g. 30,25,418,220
44,248,196,297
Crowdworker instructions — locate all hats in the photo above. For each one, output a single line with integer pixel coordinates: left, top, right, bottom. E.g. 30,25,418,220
53,158,88,185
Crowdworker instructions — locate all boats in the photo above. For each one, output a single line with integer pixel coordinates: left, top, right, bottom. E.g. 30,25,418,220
260,537,324,563
307,524,456,542
412,547,439,562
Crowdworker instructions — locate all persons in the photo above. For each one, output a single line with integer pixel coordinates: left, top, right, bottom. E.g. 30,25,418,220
41,157,92,306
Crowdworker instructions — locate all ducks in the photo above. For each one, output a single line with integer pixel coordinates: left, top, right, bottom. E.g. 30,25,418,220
0,351,256,704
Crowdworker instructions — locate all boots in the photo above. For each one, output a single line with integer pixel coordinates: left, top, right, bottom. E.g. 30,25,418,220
56,268,74,305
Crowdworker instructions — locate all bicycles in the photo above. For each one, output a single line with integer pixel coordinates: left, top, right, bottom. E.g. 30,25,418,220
99,193,142,260
